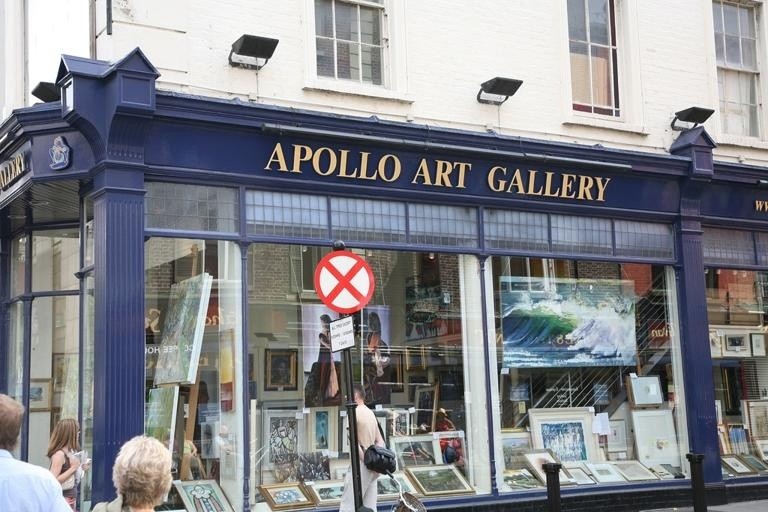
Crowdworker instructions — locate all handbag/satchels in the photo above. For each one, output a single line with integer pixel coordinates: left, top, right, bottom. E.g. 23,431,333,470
49,449,76,492
363,444,396,474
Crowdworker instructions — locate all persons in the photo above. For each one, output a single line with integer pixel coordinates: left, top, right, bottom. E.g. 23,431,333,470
430,405,457,461
363,311,391,404
269,358,292,385
90,433,172,512
0,393,75,512
339,385,387,512
47,419,93,512
311,315,341,401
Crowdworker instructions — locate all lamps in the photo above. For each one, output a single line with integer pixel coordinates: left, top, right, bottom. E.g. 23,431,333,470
227,30,280,73
665,104,716,133
475,73,525,106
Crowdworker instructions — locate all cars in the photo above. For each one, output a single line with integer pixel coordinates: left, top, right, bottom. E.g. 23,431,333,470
267,448,314,485
492,275,562,314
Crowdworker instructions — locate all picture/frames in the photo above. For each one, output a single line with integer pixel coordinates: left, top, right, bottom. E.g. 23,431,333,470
263,347,298,391
625,374,664,408
501,407,685,492
248,347,259,401
201,421,220,459
351,346,476,499
714,397,768,476
174,478,234,511
259,406,350,512
708,324,767,358
